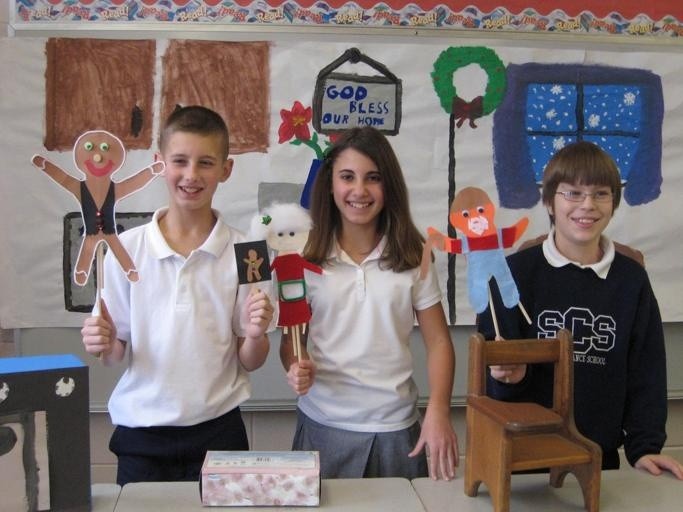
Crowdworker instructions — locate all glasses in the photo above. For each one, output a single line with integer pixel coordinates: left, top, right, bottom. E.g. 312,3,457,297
555,189,617,203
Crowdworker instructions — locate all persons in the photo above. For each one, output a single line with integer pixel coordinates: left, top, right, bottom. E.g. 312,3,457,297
278,122,461,483
79,103,279,487
473,139,683,483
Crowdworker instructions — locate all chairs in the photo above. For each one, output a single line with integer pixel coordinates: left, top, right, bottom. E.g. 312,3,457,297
463,327,601,512
